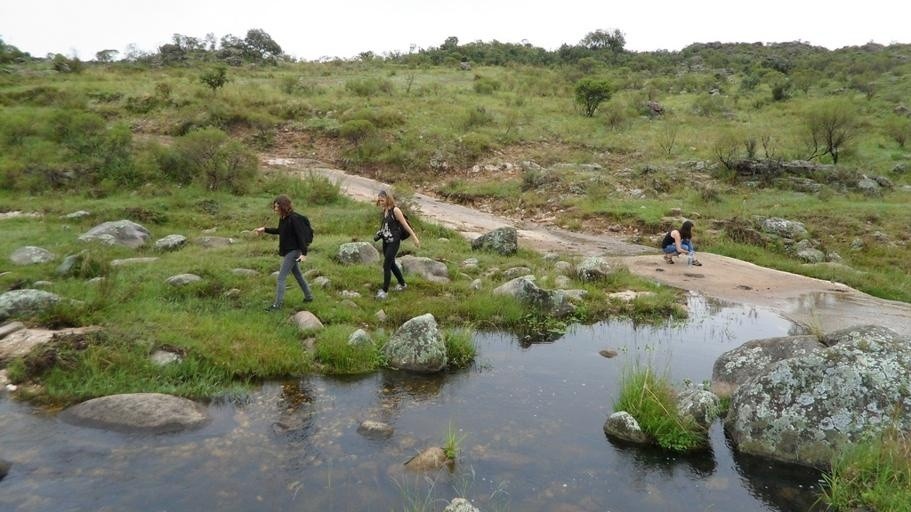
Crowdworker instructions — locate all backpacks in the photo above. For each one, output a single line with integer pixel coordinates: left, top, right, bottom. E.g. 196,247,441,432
289,213,312,244
391,206,411,240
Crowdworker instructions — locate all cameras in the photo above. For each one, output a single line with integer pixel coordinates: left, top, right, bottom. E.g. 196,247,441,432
374,231,384,241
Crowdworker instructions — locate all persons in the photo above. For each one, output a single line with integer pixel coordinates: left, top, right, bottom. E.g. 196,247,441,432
252,193,315,312
371,189,422,300
662,220,703,266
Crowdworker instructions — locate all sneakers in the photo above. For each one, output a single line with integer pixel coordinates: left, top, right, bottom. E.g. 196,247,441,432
264,304,281,313
376,291,388,298
692,260,701,266
303,298,312,304
664,255,674,264
395,282,407,292
